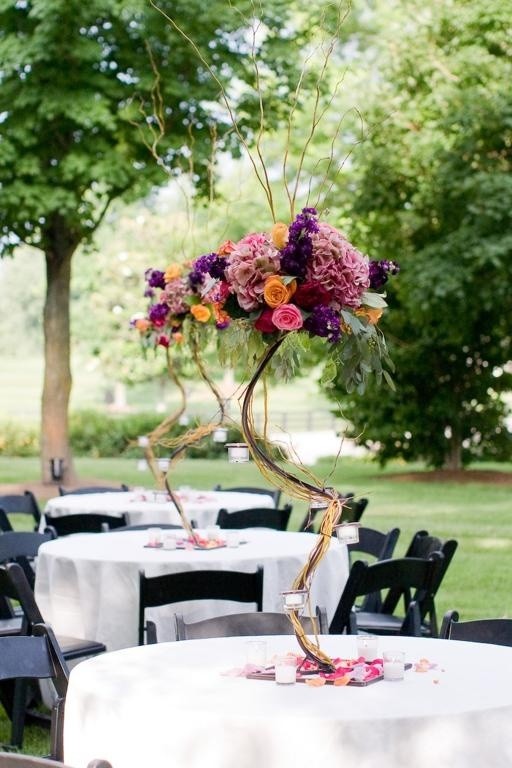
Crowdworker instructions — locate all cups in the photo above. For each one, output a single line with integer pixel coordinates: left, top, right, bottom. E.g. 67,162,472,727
132,416,249,553
356,636,379,660
277,590,308,610
275,656,297,685
246,640,265,668
383,651,405,679
